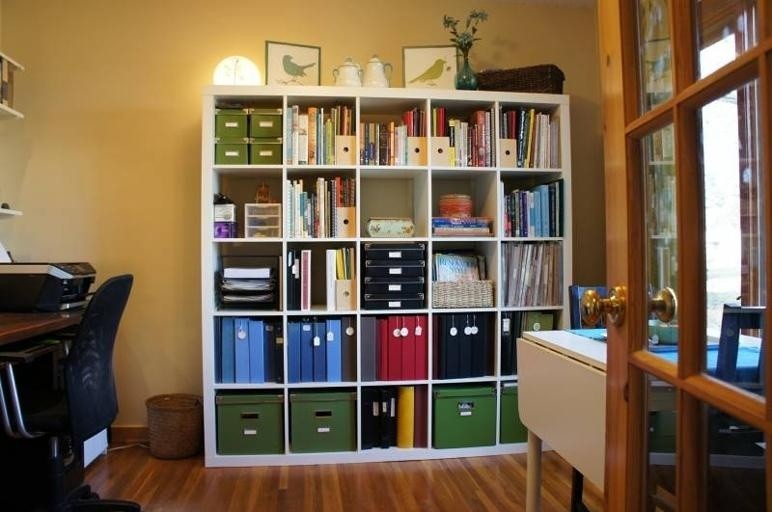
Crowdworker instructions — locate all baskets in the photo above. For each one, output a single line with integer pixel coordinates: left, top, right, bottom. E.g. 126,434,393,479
476,63,563,93
431,278,495,311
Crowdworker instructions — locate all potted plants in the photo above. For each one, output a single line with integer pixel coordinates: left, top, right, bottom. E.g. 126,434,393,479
441,6,489,89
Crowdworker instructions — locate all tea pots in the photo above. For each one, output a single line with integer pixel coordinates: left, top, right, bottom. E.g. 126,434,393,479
358,54,394,88
331,57,362,87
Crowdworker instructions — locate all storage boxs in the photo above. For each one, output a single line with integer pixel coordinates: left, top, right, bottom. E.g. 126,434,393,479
214,222,237,238
367,215,415,239
287,392,357,453
213,136,249,165
500,382,528,444
214,395,283,455
216,109,250,139
432,385,497,450
247,137,283,164
215,204,237,222
248,107,283,138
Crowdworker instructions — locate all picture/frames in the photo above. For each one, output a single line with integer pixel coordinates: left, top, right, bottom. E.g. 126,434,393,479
401,45,459,87
265,39,321,85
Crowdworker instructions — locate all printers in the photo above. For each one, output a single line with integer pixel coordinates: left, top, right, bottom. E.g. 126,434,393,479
0,250,97,315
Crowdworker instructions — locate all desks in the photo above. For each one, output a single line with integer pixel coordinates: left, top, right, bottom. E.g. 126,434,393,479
517,329,762,512
0,306,87,343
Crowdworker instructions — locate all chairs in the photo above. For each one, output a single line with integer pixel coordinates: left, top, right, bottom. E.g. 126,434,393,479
563,284,613,512
1,271,142,511
705,303,767,459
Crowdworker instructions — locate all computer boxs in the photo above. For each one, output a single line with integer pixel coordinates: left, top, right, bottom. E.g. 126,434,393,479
64,338,110,470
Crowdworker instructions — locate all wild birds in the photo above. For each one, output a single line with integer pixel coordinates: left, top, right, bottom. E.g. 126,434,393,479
282,54,316,77
408,58,447,84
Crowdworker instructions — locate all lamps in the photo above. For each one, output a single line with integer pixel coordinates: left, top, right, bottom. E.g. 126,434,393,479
210,54,261,83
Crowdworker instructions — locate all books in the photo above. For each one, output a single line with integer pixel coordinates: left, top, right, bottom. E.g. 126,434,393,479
286,103,558,168
500,178,564,237
433,248,487,281
502,242,563,308
287,176,356,238
288,246,356,311
432,216,496,237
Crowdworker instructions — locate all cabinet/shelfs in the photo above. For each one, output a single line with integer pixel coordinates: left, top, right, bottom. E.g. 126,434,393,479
202,84,575,472
0,52,25,216
244,203,282,237
